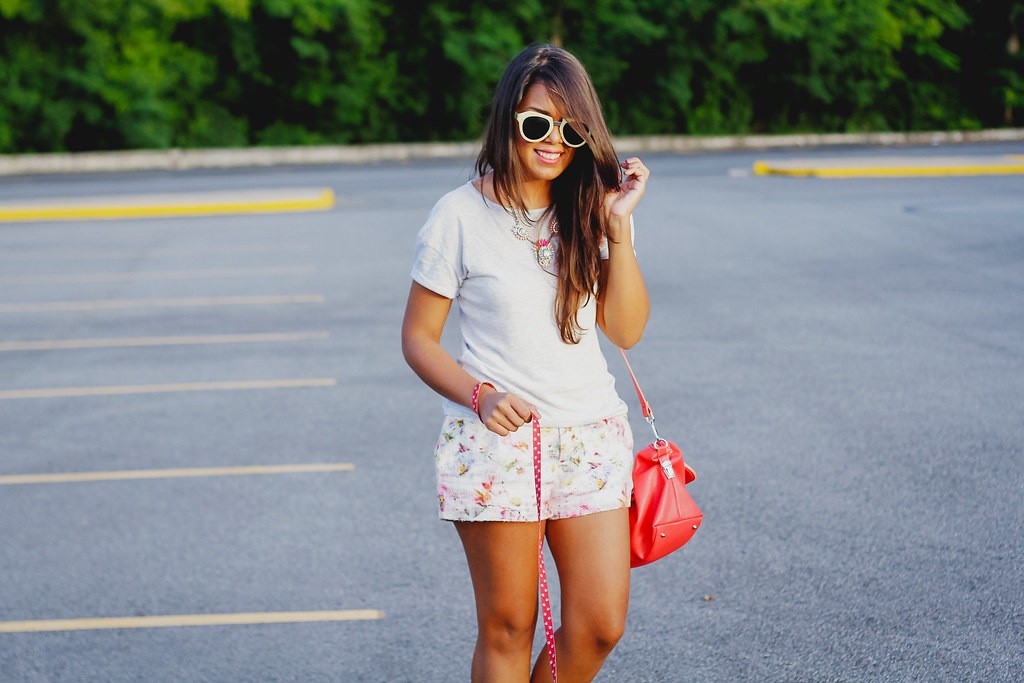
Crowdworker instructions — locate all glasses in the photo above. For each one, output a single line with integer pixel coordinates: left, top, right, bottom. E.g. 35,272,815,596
510,109,591,148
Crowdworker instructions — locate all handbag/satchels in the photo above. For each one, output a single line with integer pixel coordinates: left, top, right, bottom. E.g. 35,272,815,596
630,439,703,568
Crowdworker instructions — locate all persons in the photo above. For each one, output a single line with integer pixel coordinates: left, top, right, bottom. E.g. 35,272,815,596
401,45,652,683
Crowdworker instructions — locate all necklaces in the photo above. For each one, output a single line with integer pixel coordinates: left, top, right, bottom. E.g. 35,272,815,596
506,195,560,265
